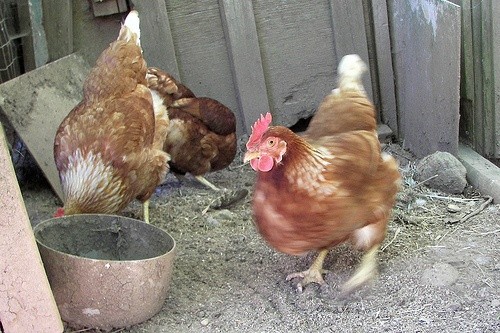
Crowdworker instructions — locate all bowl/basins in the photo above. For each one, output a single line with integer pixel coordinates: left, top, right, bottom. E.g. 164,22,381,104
33,213,176,327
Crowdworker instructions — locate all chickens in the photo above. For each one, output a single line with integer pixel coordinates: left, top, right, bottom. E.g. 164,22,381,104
243,53,400,293
52,10,171,225
145,62,236,194
